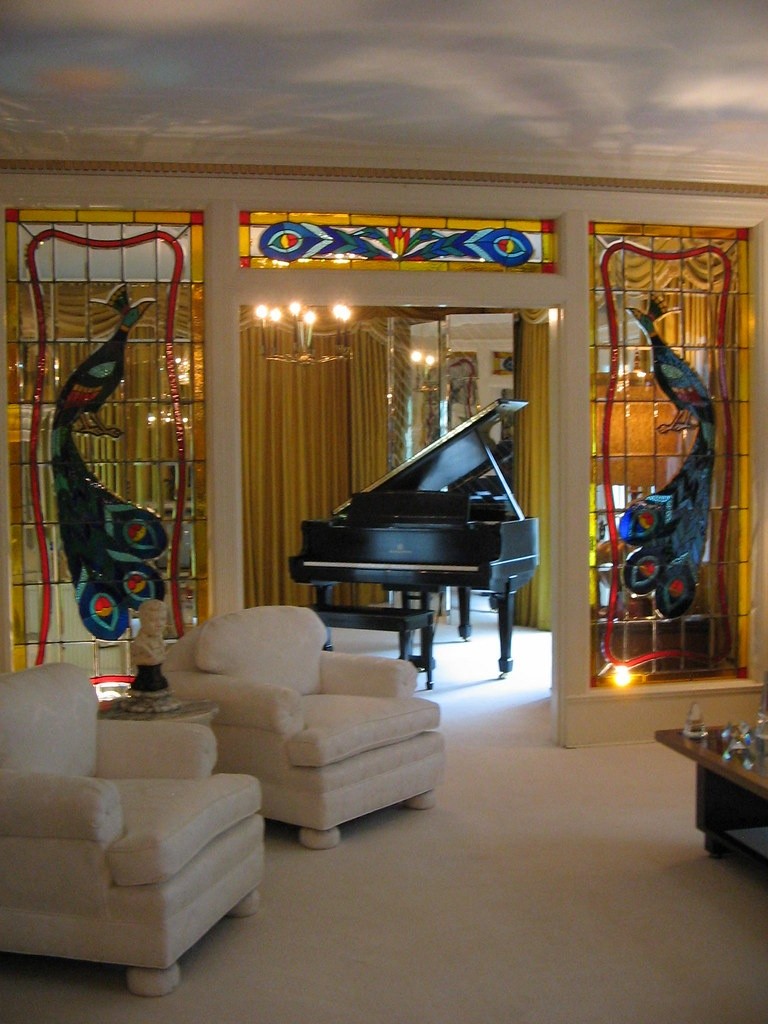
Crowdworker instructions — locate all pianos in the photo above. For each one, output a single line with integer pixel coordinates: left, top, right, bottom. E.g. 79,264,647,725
288,396,540,692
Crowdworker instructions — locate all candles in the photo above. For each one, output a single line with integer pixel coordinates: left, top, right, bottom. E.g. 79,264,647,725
333,305,350,347
412,351,423,389
255,305,267,345
270,308,281,347
290,301,314,347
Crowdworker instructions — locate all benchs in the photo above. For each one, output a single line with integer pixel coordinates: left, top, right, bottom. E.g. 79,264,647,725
303,609,435,690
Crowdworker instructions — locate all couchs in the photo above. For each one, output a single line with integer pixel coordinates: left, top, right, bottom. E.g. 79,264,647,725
159,606,441,851
0,662,266,998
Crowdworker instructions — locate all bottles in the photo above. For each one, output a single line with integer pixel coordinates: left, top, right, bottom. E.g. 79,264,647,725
755,670,768,777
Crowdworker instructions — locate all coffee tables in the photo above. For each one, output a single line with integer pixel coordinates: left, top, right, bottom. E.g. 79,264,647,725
104,694,220,725
653,723,768,873
